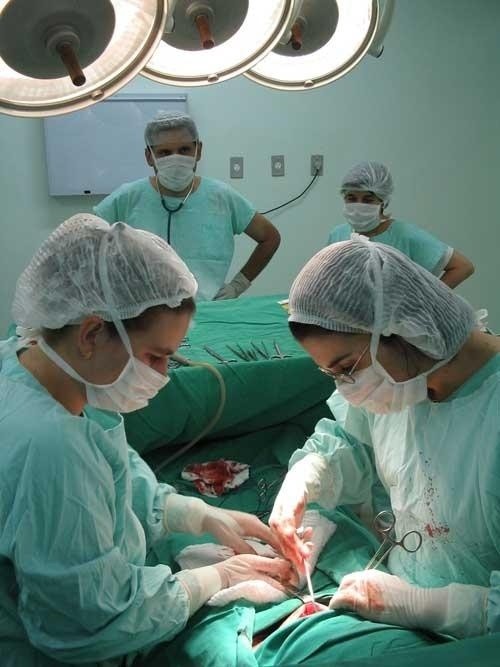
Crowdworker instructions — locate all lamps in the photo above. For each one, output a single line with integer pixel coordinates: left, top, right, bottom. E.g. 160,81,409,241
241,1,381,94
137,1,295,88
0,1,171,121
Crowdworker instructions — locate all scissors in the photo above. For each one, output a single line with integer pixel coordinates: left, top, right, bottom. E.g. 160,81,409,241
363,510,423,571
257,478,281,514
202,339,294,364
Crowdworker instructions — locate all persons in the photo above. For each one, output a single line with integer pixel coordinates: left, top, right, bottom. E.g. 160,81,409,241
92,110,281,301
268,232,500,640
327,160,475,288
1,212,300,667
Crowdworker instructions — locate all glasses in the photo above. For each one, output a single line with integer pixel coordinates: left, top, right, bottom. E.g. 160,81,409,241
316,347,370,385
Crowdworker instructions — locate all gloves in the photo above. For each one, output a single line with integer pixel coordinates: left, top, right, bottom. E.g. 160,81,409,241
263,452,329,563
216,272,252,301
165,492,281,555
328,567,492,639
176,549,286,612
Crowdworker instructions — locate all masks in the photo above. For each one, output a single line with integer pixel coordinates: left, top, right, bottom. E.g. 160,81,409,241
51,317,169,416
149,144,202,193
333,316,436,419
342,201,386,234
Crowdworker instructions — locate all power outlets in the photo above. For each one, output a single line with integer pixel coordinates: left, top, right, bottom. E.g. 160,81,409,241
310,154,323,176
271,155,284,176
230,157,243,178
